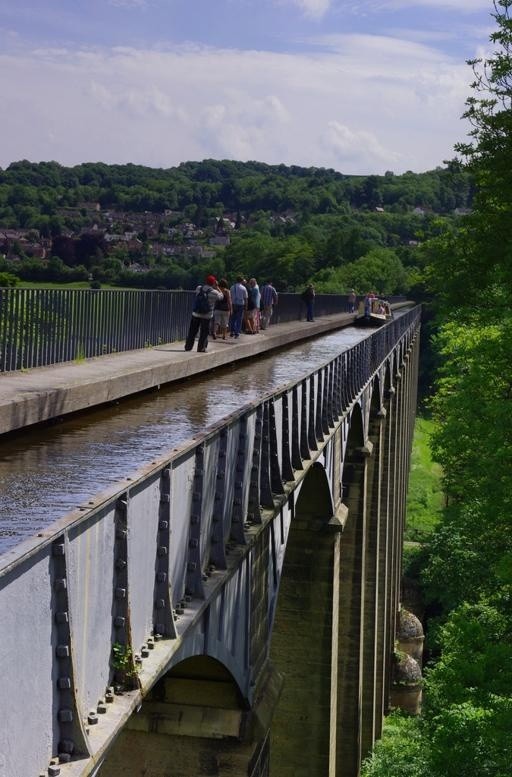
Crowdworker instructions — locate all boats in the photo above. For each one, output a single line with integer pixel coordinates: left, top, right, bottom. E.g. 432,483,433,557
354,312,393,325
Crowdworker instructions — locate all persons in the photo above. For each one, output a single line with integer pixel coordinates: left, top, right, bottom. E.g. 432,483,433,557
303,284,316,322
184,275,278,353
347,289,392,315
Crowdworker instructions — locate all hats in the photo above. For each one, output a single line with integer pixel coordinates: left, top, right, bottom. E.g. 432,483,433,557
306,284,314,289
206,275,216,283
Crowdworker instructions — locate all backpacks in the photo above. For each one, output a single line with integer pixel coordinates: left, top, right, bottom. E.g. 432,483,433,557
214,294,230,312
192,286,214,314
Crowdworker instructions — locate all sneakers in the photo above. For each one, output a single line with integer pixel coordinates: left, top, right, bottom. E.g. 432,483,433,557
260,327,266,330
197,348,205,352
230,334,239,339
307,320,314,322
246,330,259,335
223,336,225,339
185,347,192,351
212,333,217,340
